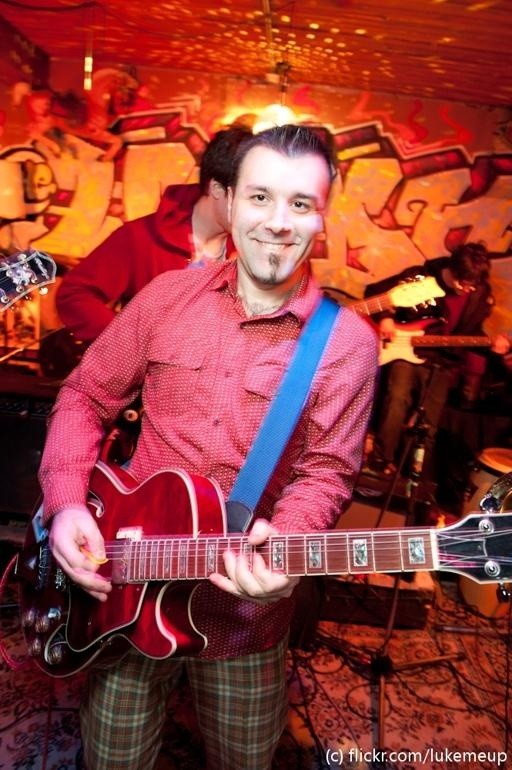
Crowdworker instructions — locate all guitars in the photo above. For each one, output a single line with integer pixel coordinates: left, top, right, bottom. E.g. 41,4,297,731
2,253,58,311
18,459,510,679
377,318,507,368
347,274,446,317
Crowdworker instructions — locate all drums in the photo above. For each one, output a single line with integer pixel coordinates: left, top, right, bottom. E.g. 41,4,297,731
459,450,511,619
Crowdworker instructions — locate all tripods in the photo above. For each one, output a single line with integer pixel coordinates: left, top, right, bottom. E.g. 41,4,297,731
328,575,463,770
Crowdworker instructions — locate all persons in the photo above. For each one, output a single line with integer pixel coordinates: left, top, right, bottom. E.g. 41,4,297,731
36,123,381,769
55,125,257,347
362,243,511,473
